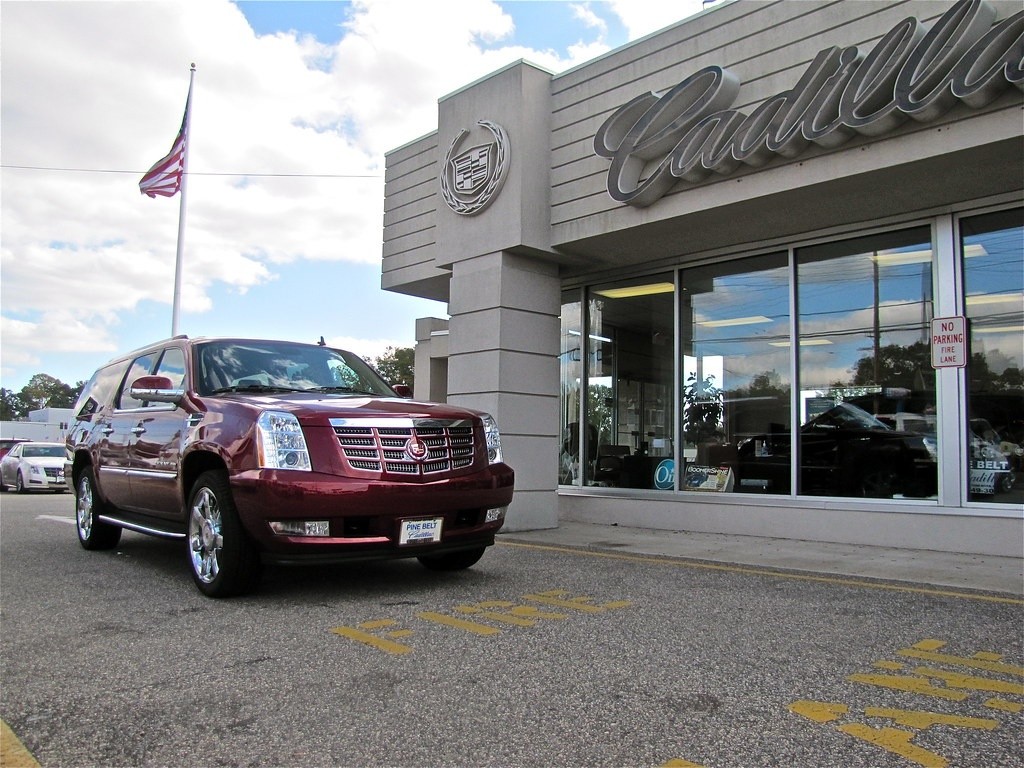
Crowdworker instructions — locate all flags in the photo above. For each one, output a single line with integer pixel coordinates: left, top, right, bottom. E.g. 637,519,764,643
138,82,191,199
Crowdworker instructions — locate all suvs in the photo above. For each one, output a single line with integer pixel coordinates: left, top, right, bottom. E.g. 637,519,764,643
64,335,516,601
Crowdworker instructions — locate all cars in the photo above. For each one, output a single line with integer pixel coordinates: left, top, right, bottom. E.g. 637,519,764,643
0,438,33,461
678,383,1024,498
0,442,74,492
